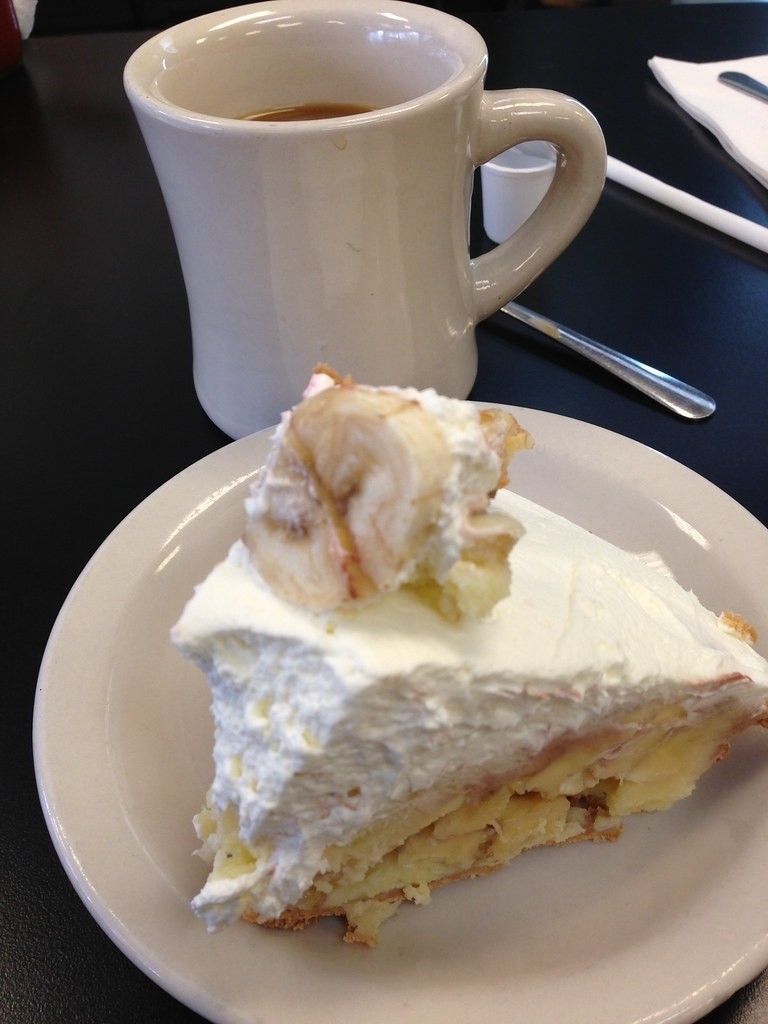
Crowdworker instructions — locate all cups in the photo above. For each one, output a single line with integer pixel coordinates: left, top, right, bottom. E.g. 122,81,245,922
124,0,609,439
481,140,556,244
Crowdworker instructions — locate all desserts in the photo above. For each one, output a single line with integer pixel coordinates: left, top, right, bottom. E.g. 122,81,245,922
169,362,768,946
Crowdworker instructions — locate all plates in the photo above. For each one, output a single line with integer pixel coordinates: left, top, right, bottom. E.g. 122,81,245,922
649,56,768,190
32,404,768,1023
